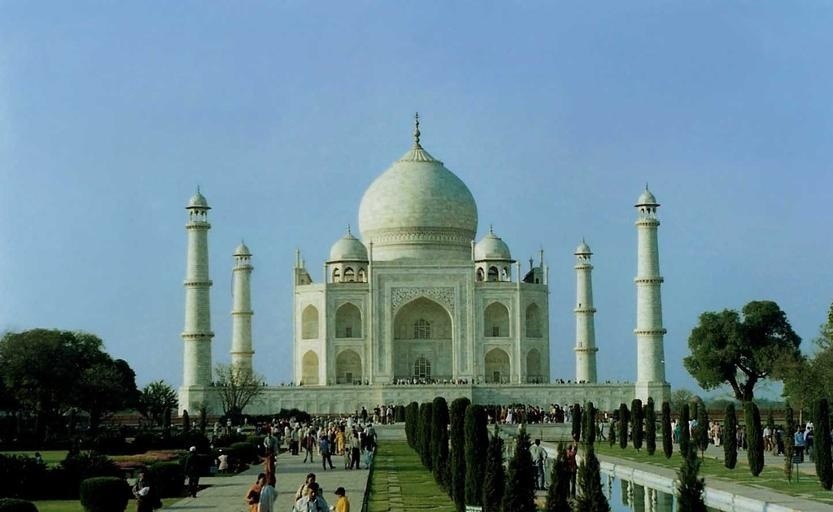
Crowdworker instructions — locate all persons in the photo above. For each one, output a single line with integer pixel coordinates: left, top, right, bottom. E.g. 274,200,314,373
395,376,468,385
34,451,42,464
598,419,606,440
562,438,578,497
185,445,201,498
482,403,574,424
212,403,395,511
131,468,153,511
529,438,547,490
669,416,815,463
